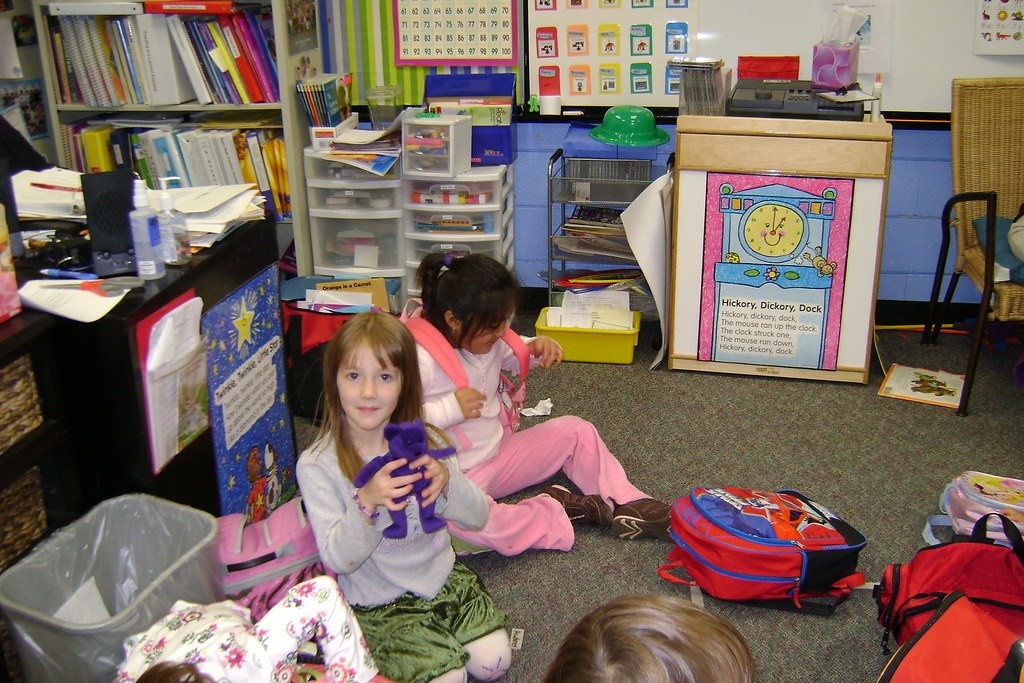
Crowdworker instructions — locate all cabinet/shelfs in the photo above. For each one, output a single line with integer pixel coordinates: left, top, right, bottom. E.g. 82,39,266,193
13,208,299,534
303,115,515,315
31,0,324,278
548,148,676,350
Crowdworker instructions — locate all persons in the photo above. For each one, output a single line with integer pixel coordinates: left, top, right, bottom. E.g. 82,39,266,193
296,313,512,683
540,594,753,683
402,252,673,555
119,575,378,683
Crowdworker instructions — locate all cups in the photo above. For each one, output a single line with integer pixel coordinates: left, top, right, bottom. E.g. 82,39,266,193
539,96,562,115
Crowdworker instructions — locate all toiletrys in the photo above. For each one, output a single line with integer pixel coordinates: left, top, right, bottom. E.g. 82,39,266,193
130,176,194,279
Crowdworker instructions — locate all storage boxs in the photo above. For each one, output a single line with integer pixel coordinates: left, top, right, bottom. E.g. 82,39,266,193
535,308,640,364
309,112,360,151
421,72,518,166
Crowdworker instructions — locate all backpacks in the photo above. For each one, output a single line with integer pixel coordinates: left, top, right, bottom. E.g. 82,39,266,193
922,472,1024,543
656,487,870,615
216,500,339,624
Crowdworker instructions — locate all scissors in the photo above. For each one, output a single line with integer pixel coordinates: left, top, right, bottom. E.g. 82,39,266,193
41,276,145,297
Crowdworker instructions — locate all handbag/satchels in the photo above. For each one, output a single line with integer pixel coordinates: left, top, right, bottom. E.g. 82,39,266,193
875,509,1024,657
283,300,404,421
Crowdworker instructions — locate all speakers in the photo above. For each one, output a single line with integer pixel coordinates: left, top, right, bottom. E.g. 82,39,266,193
80,169,137,278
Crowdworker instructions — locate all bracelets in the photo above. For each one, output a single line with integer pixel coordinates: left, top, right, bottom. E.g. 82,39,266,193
353,488,381,520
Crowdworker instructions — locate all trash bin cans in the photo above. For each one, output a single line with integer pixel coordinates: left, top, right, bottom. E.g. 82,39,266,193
0,492,221,683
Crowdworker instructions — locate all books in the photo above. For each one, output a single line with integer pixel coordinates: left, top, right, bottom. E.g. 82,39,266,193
58,11,291,221
297,73,351,126
561,205,632,251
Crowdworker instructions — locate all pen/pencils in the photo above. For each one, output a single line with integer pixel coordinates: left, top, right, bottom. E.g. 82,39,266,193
31,183,83,192
37,269,99,280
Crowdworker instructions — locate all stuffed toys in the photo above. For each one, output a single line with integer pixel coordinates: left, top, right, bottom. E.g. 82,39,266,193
353,417,456,540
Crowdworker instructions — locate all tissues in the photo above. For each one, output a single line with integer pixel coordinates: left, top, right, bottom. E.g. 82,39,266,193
811,5,870,88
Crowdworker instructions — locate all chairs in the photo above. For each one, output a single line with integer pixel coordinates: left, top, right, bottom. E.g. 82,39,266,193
920,77,1024,417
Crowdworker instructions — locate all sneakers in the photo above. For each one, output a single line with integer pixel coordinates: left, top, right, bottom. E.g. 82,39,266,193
540,484,616,525
612,497,677,544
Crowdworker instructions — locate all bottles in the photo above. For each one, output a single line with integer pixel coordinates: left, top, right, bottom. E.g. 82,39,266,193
128,179,166,279
365,85,404,130
870,73,882,122
